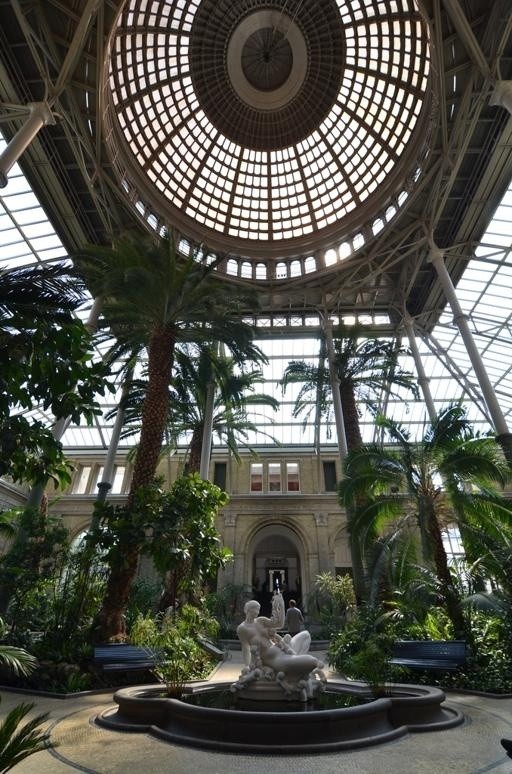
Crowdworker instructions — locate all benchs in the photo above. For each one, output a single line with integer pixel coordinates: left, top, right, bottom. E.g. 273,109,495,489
385,639,469,683
92,641,185,690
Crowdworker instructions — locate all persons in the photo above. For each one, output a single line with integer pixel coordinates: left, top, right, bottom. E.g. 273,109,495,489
285,599,304,638
236,593,318,684
